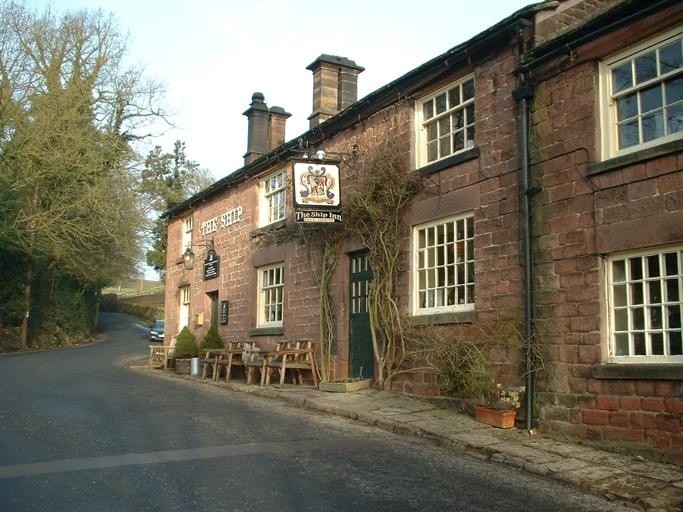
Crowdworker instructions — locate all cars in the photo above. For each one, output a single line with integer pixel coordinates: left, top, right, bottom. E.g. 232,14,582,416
149,319,164,343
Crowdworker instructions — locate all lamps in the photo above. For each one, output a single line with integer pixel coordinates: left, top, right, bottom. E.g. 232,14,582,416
181,237,215,270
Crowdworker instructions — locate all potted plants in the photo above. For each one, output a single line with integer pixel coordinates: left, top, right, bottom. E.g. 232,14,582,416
173,324,198,373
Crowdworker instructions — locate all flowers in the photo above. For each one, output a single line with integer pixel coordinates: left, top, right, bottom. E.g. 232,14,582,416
490,383,525,410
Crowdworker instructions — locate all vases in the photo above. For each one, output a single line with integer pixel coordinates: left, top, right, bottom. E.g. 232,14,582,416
474,403,516,428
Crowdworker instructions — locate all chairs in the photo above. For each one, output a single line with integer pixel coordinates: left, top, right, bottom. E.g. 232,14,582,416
147,334,178,372
198,339,319,388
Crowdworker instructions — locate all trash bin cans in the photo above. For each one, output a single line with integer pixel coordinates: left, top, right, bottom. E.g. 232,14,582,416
191,358,201,376
